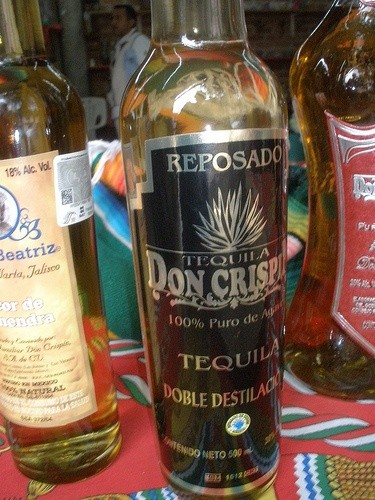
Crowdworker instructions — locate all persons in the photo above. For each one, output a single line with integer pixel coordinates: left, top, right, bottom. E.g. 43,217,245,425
104,3,151,141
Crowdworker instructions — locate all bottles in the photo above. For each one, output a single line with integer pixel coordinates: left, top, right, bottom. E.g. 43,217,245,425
119,0,287,500
0,1,123,484
286,0,375,399
